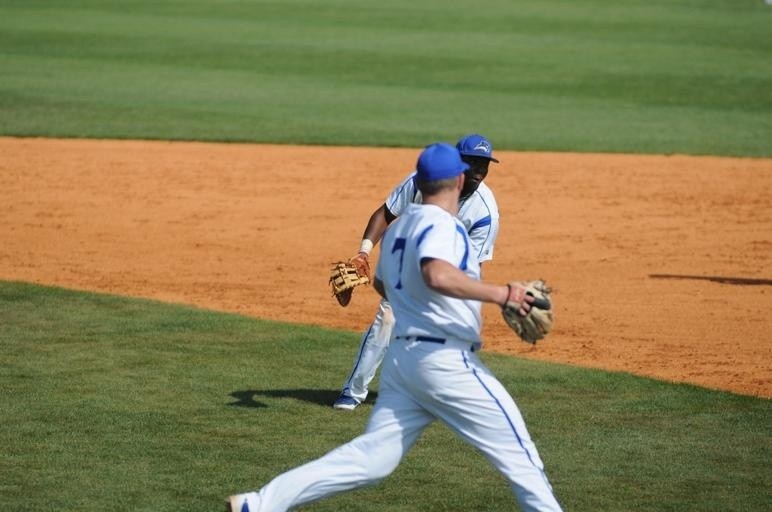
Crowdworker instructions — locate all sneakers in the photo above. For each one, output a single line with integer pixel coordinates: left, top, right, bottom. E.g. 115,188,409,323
229,492,260,512
333,395,359,410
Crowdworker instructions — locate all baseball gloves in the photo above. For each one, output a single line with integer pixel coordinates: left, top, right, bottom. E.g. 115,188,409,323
331,255,371,307
503,280,553,344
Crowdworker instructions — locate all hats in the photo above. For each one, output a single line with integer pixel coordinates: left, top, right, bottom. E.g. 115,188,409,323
455,134,500,164
416,142,469,180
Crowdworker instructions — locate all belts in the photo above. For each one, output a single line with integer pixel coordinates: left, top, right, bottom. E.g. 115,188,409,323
395,336,446,344
